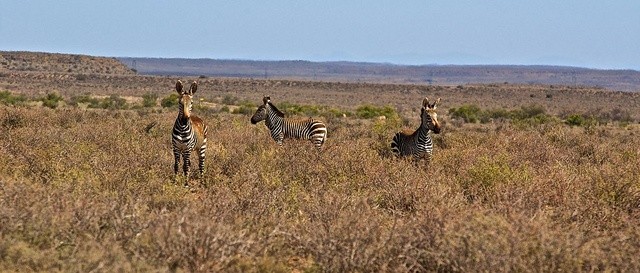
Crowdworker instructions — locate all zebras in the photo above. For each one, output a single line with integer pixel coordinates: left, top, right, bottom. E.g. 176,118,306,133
171,78,208,188
390,95,442,168
251,94,328,153
